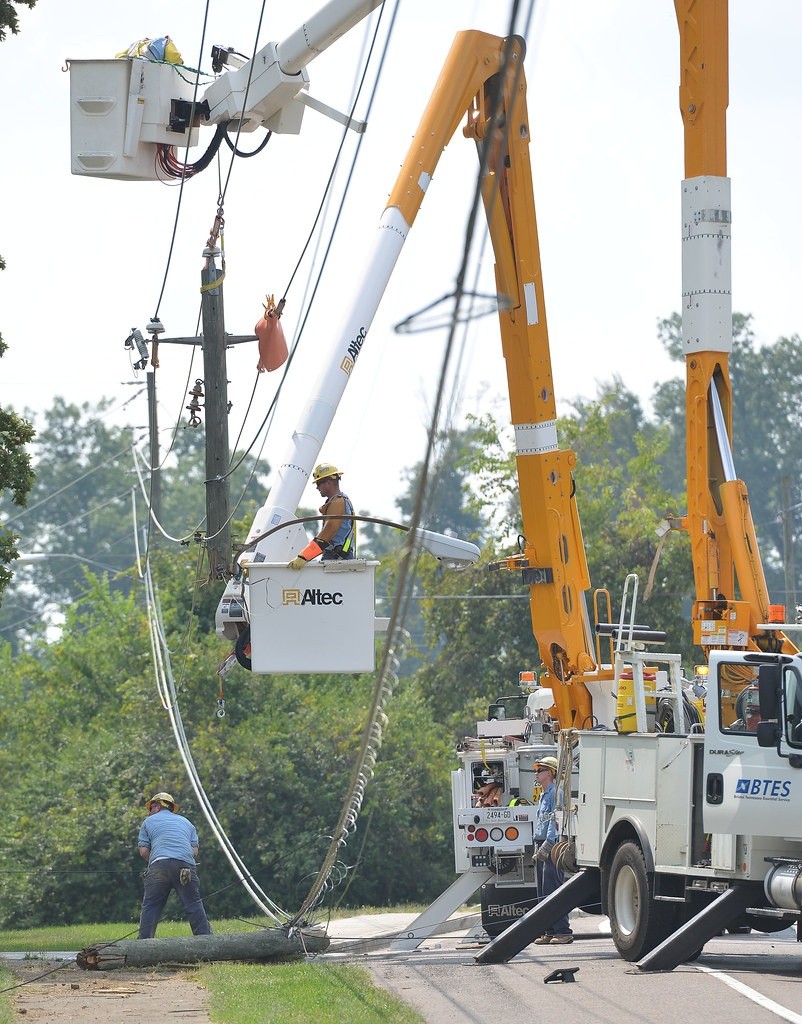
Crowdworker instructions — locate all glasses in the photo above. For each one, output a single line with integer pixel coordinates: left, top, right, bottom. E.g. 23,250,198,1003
316,481,327,487
150,805,157,812
537,768,548,773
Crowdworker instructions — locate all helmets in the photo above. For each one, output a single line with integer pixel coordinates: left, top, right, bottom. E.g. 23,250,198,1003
533,756,558,775
313,463,343,483
146,792,178,813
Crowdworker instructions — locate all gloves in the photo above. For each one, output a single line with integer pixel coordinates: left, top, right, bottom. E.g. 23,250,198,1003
180,868,192,886
536,849,549,861
287,557,306,570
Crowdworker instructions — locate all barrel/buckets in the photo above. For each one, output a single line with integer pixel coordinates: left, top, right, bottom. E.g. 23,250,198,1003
616,672,656,732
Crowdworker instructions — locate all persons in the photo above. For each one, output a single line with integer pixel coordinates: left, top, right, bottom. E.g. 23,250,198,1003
529,756,574,945
287,462,356,571
137,792,213,939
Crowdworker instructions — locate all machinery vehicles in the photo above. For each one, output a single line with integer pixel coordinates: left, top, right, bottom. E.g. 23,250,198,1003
57,2,802,990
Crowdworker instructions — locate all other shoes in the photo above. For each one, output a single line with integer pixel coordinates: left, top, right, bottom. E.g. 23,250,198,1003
534,935,551,944
549,935,573,944
